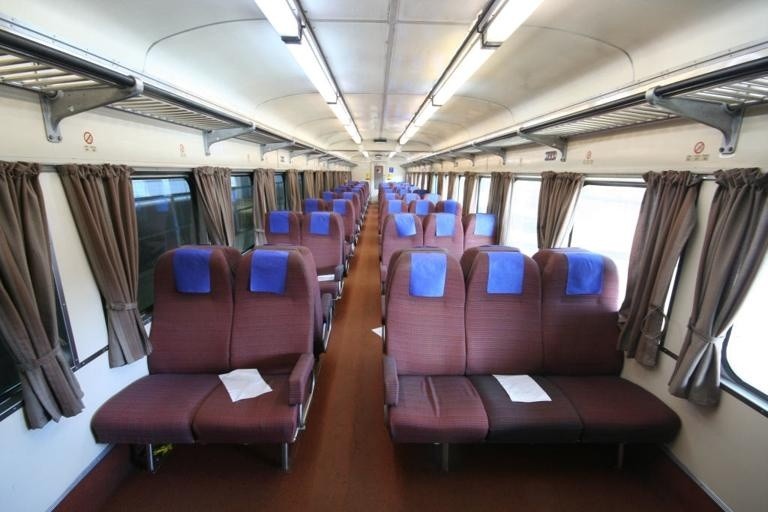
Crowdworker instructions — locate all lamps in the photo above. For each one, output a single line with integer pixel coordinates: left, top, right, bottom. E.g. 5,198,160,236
253,0,540,158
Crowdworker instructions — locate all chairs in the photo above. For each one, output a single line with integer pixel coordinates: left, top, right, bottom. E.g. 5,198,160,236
93,244,237,473
373,248,493,473
193,247,322,469
464,247,587,472
373,179,505,329
547,249,680,469
247,180,373,352
